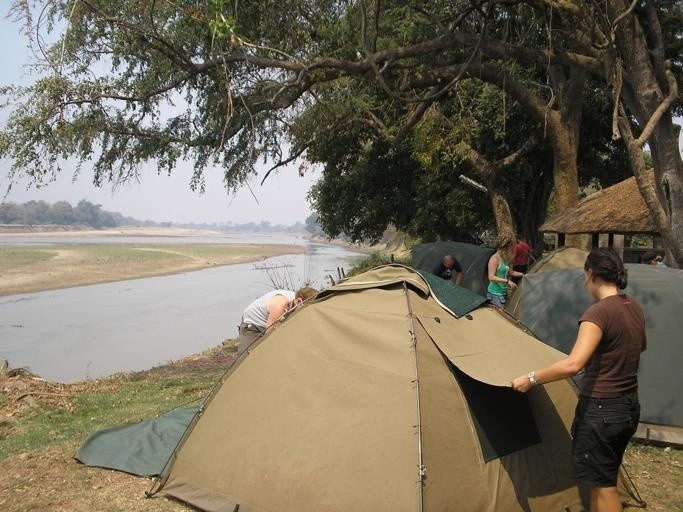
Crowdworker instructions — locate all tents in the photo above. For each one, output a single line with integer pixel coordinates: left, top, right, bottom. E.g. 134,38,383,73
404,242,499,305
161,258,645,510
513,262,683,447
498,242,592,331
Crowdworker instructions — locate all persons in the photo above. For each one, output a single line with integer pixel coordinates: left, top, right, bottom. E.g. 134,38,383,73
642,250,667,268
506,234,531,273
237,286,321,357
432,255,464,286
653,249,669,267
486,243,523,310
510,246,648,510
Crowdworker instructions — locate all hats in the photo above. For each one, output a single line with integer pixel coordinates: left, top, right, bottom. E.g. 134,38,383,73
495,235,511,248
641,251,657,264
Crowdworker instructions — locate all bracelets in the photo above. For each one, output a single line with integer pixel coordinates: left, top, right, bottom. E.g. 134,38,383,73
527,371,537,385
507,279,509,283
